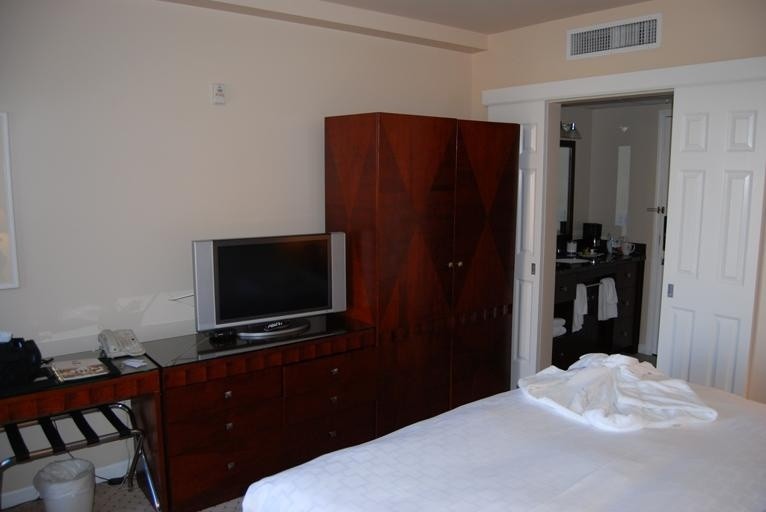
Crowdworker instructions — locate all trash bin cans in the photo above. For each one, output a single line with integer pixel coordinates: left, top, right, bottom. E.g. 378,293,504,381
32,458,96,512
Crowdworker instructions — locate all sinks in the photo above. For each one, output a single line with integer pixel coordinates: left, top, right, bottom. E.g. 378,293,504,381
556,257,592,264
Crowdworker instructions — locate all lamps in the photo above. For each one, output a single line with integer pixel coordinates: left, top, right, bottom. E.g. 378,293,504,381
560,121,583,139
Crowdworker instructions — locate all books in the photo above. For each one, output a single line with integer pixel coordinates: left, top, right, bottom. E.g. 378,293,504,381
51,357,108,381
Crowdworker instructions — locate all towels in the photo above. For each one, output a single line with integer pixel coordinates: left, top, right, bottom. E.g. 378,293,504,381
572,277,620,332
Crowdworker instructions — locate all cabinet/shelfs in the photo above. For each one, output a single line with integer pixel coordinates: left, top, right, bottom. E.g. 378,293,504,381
552,238,646,370
140,315,374,512
323,112,520,463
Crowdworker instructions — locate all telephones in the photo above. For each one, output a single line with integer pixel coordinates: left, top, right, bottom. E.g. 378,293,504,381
96,327,145,359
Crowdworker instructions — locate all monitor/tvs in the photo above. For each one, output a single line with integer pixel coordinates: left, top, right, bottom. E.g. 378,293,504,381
193,232,347,337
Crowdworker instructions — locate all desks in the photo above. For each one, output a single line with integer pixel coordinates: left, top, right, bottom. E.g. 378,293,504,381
0,346,168,512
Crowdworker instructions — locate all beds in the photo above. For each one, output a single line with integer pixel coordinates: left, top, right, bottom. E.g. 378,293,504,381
243,352,766,511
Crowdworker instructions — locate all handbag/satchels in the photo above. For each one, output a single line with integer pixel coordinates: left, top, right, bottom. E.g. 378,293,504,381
1,337,42,396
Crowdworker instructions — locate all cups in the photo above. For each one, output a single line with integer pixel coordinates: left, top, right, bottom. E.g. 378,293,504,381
565,241,578,257
622,243,635,256
